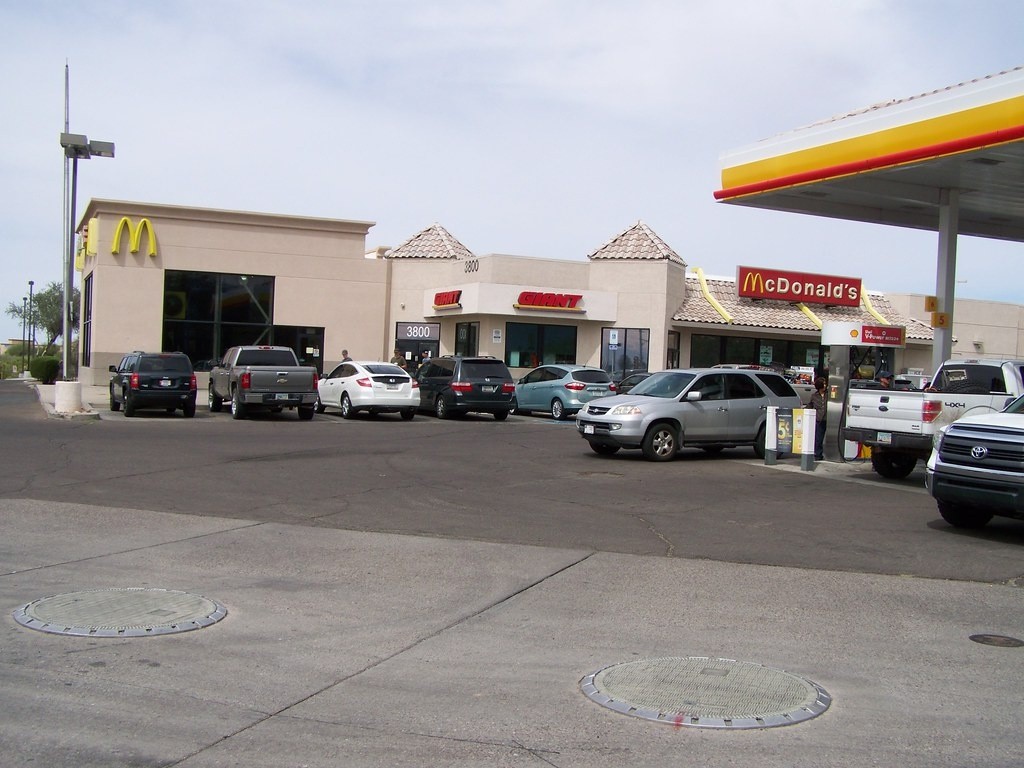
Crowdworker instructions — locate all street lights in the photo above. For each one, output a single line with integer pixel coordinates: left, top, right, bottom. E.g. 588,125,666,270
53,133,117,417
28,280,36,371
22,297,28,375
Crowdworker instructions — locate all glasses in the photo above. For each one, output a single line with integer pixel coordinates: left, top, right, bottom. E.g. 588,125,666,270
886,377,892,380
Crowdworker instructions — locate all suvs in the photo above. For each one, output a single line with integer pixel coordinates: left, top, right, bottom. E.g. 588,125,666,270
412,354,515,420
107,350,198,418
925,393,1023,525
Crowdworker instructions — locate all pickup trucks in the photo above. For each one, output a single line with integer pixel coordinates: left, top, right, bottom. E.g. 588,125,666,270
711,364,817,406
849,378,894,390
208,346,318,420
848,358,1024,483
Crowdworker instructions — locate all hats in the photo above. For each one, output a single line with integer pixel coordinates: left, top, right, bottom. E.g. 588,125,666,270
394,349,400,353
880,371,895,378
342,350,348,354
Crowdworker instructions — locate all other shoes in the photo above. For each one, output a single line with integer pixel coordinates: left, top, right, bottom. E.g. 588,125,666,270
816,455,823,460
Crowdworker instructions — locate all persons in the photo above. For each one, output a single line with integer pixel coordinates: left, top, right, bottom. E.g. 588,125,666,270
391,349,407,369
341,350,353,363
801,377,829,460
877,372,895,390
420,352,431,364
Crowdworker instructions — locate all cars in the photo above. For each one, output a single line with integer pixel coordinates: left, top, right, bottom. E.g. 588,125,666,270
888,378,918,390
509,364,617,419
615,372,671,395
315,361,422,420
575,368,803,461
